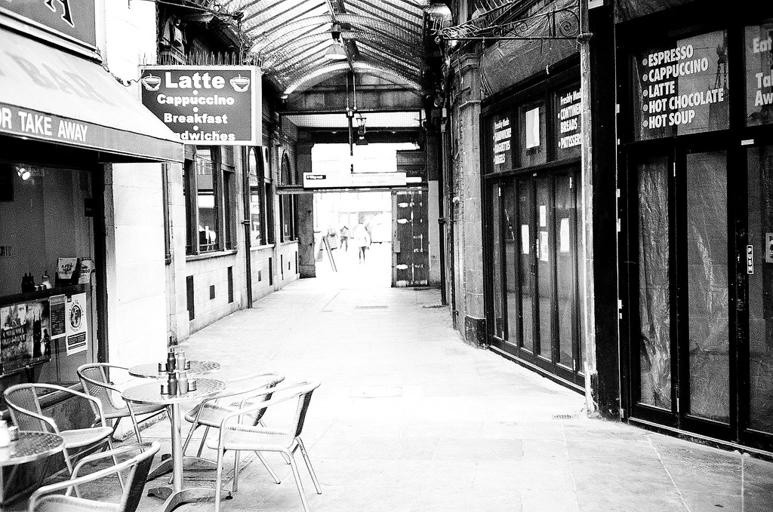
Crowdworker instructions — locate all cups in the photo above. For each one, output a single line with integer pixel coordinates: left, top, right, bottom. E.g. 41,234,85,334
229,77,250,93
142,76,162,92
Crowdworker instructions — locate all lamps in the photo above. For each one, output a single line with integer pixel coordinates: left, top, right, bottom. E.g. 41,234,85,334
325,22,347,60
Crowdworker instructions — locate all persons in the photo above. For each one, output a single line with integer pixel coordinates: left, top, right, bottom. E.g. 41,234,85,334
339,222,371,262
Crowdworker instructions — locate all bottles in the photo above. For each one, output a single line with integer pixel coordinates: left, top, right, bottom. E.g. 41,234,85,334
21,271,53,293
158,329,196,396
0,420,19,448
